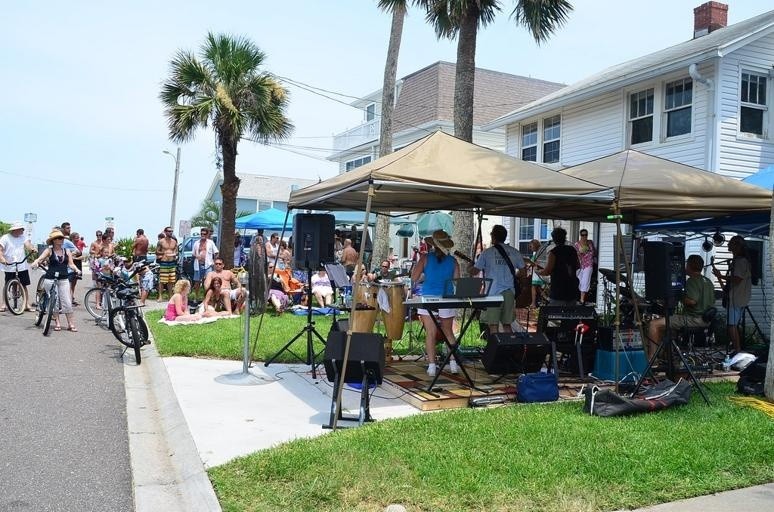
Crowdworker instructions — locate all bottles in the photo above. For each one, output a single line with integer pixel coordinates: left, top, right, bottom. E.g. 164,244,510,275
723,354,730,373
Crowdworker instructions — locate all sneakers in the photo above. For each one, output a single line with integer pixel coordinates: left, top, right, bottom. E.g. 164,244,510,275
450,365,460,374
427,370,436,376
526,305,536,308
650,359,659,368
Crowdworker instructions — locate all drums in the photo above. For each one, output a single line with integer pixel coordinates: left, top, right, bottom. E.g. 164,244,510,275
383,338,393,367
379,283,406,341
651,297,675,315
628,302,658,320
351,281,379,334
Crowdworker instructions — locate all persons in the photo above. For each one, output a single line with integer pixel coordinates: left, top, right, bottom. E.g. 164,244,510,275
28,222,597,332
410,229,461,377
647,254,716,371
465,225,528,339
711,235,752,356
0,220,38,312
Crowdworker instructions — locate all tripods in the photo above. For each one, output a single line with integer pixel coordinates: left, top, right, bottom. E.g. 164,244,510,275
393,307,427,360
264,276,327,378
739,306,768,347
631,314,712,407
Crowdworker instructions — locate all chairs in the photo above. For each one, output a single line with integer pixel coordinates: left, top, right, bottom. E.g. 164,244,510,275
682,307,717,376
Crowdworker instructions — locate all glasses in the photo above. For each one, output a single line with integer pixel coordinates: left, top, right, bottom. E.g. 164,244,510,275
216,263,222,265
581,234,587,236
55,237,63,240
167,231,173,232
209,230,213,232
382,266,387,269
97,235,101,236
201,233,206,235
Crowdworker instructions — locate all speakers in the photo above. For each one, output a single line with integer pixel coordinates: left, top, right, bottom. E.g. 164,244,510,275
292,213,334,271
482,332,545,374
744,239,763,280
324,329,385,384
644,240,687,298
330,318,349,330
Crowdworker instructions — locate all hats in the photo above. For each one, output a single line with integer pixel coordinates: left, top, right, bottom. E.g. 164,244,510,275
8,222,25,231
424,231,455,256
46,230,69,245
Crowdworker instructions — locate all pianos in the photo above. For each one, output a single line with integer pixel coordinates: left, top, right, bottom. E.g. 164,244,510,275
401,296,505,309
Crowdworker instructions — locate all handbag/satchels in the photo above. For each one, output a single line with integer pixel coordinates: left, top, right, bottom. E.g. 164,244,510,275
516,371,560,403
582,377,692,417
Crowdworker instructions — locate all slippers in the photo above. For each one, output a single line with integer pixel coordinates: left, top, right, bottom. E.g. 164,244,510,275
24,307,35,312
54,325,62,331
67,327,78,332
0,307,6,312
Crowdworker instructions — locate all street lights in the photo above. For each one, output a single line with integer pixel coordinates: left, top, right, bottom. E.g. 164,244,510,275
162,147,181,233
24,211,38,245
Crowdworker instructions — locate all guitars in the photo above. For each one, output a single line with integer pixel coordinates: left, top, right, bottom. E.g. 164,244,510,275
710,256,727,307
523,256,581,300
454,251,521,298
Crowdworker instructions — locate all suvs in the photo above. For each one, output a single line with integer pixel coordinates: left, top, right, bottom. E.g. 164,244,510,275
146,234,269,293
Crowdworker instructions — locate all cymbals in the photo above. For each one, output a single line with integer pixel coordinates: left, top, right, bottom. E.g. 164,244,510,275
598,267,628,282
613,287,641,298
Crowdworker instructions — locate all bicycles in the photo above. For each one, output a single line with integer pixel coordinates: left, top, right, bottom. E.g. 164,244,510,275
84,269,121,333
96,265,149,363
31,262,83,336
4,250,36,315
